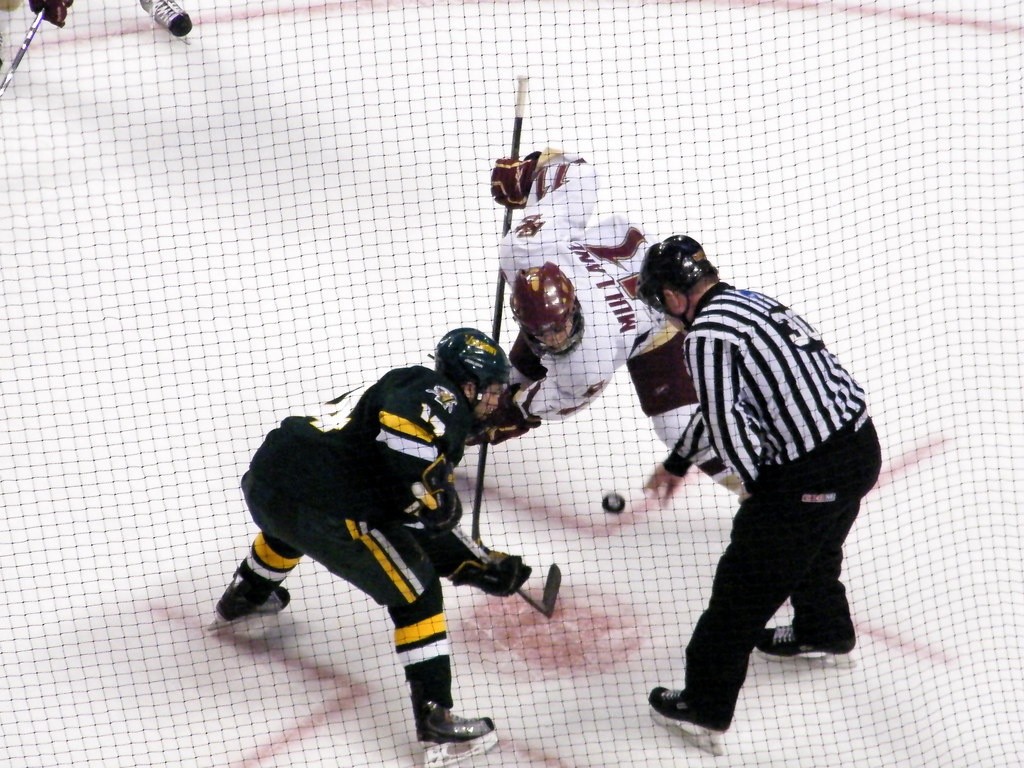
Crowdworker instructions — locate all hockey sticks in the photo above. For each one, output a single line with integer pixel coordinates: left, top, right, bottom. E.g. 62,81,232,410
410,480,563,620
469,71,534,563
0,7,46,98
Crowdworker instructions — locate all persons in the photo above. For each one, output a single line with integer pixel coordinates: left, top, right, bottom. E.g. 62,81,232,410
465,148,749,502
638,235,882,731
218,328,532,742
0,0,192,67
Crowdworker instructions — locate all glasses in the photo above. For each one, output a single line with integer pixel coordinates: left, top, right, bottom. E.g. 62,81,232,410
534,325,567,338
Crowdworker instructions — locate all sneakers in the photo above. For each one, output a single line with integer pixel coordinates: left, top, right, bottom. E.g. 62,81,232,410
206,565,290,631
752,624,857,661
139,0,192,45
646,686,730,736
409,695,499,768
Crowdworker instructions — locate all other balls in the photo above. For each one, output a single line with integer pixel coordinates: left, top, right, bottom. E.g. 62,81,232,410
602,493,626,513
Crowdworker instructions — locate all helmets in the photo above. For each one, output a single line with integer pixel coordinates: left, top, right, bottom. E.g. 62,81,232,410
635,235,712,314
433,327,511,446
510,261,585,360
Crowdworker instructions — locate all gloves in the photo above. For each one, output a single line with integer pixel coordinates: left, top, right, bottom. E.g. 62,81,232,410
490,158,537,210
446,537,532,597
464,382,542,445
28,0,74,28
